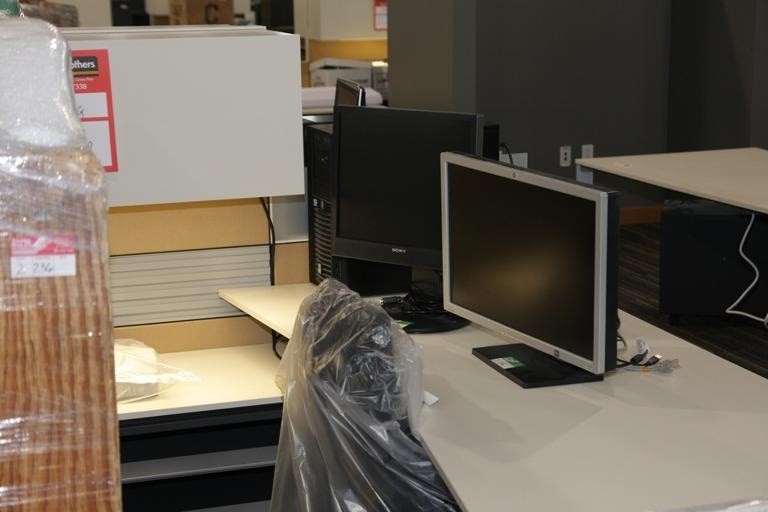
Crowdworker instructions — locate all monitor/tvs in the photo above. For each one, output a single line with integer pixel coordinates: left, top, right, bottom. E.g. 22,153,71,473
334,79,365,112
331,104,485,334
440,148,620,389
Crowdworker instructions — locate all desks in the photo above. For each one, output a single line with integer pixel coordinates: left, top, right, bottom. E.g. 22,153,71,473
571,147,768,217
114,340,294,510
217,283,767,509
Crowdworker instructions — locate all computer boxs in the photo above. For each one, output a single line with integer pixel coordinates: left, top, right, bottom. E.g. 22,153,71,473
306,124,412,296
303,114,334,166
